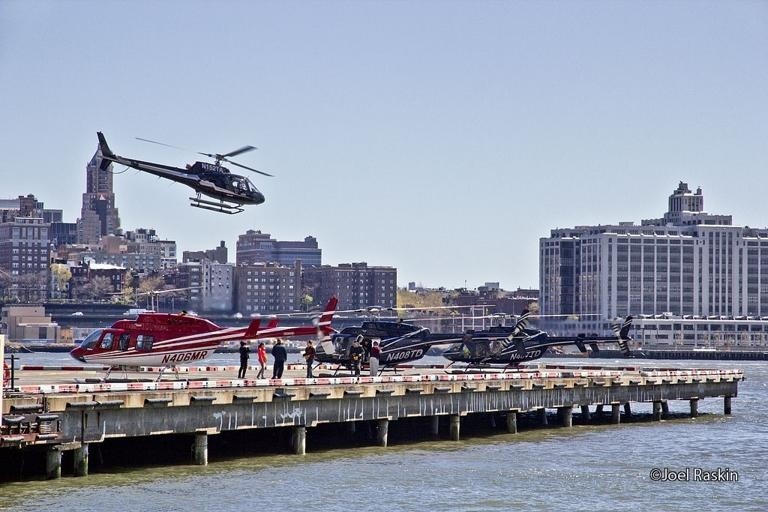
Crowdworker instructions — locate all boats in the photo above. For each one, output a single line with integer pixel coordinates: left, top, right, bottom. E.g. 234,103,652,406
212,334,306,356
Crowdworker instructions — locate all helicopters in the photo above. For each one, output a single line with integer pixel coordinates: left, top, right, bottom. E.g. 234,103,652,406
277,295,535,378
73,281,345,385
93,126,274,217
441,298,636,377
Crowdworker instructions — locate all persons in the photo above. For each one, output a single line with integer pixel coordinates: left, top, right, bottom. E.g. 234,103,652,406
3,360,11,385
238,340,251,379
272,337,288,379
349,340,366,376
302,339,315,378
335,337,346,353
256,341,268,380
369,341,382,376
